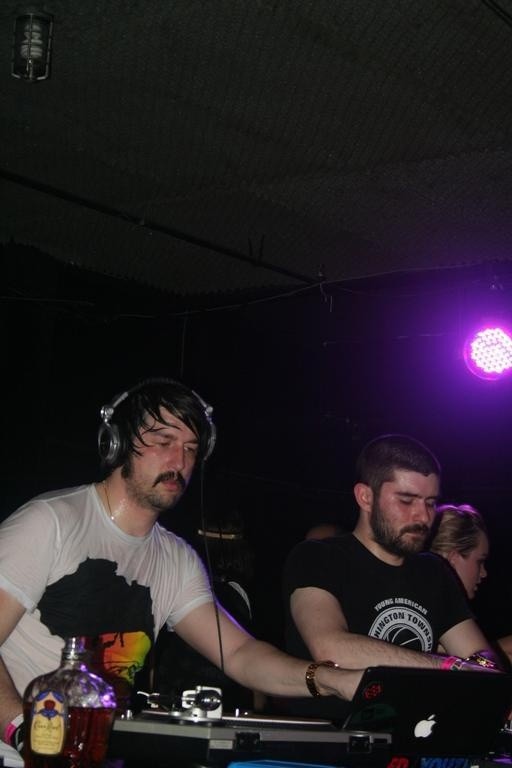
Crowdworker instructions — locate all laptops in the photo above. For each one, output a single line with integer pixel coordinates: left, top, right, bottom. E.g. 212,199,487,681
344,665,512,758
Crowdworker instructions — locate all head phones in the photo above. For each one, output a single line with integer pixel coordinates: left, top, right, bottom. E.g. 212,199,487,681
97,378,218,464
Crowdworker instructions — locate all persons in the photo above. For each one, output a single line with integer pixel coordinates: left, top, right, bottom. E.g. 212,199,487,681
286,433,511,722
1,376,367,766
425,504,510,661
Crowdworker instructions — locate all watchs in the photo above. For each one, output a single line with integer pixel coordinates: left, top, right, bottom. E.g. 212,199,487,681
305,660,337,700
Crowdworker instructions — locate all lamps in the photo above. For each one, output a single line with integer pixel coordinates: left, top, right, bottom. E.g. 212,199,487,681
459,319,510,383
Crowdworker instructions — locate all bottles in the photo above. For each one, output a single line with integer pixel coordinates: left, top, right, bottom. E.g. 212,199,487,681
22,636,116,767
